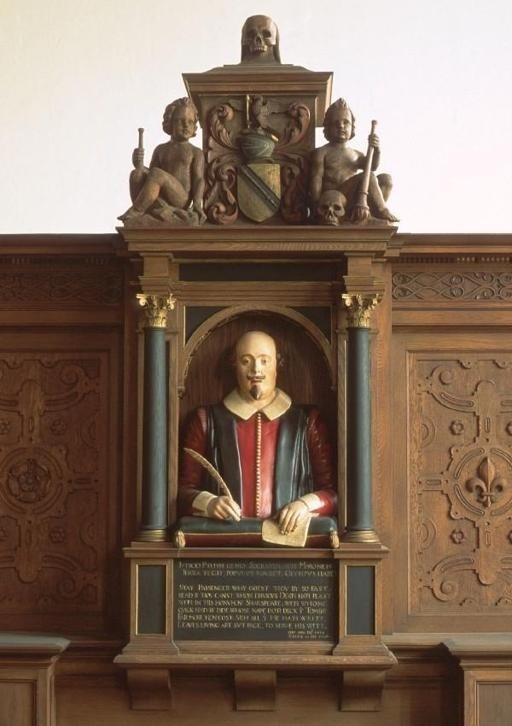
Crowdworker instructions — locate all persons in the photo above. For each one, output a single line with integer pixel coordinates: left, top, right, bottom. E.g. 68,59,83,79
310,98,400,223
117,98,207,226
179,330,338,536
